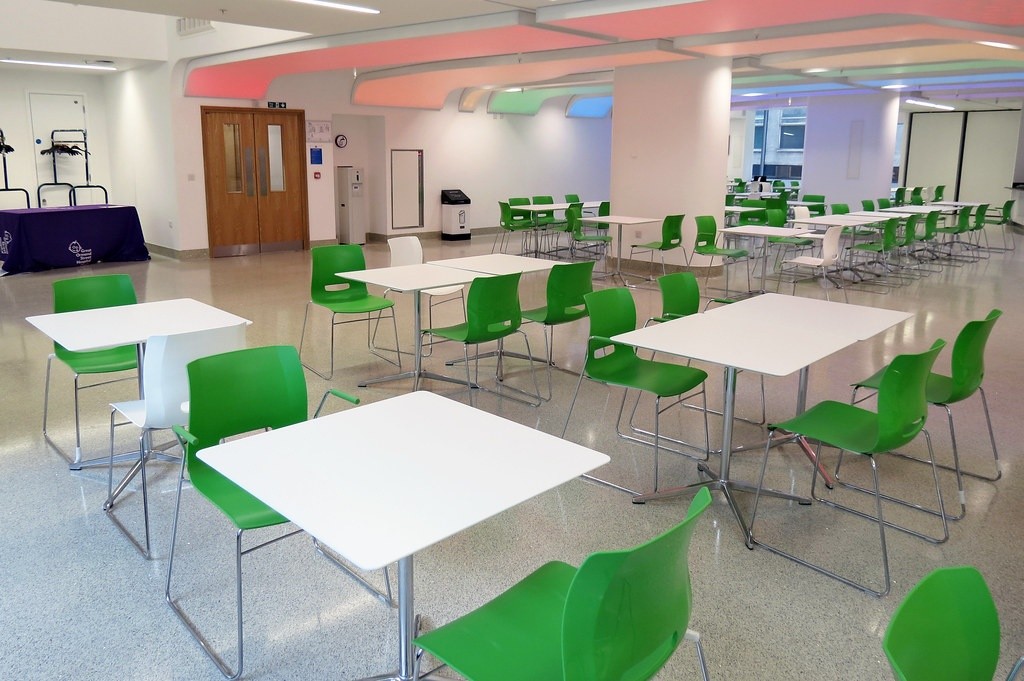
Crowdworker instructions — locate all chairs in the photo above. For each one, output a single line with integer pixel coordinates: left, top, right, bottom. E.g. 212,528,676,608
41,178,1024,681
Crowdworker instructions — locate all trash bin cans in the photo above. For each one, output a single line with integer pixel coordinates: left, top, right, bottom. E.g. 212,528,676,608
441,190,471,241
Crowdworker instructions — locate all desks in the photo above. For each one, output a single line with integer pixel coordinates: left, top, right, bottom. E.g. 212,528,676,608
716,225,817,301
877,205,964,265
0,204,151,278
923,201,989,251
334,263,499,393
785,217,875,289
196,386,611,681
509,201,606,259
576,215,662,287
609,312,859,550
731,193,781,199
25,298,254,512
787,200,824,207
815,214,891,283
703,293,914,488
845,211,917,273
427,253,575,382
723,206,766,249
